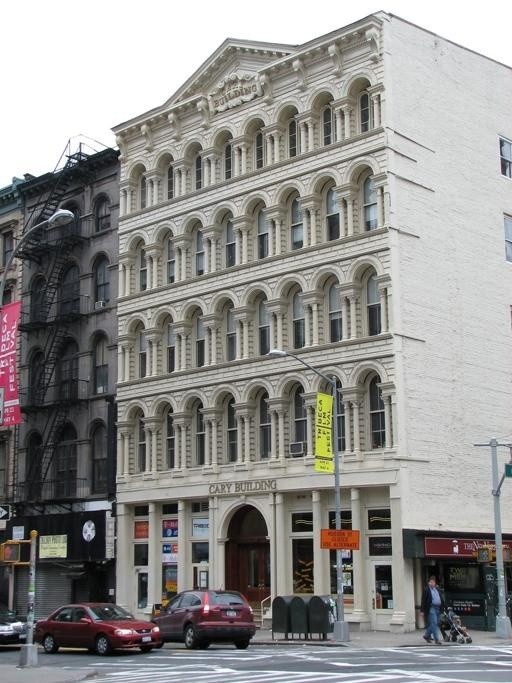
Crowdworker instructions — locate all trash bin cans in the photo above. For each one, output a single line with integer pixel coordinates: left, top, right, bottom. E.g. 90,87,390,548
152,603,161,617
272,595,334,640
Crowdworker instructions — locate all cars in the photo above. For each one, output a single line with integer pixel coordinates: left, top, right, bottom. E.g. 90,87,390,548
1,608,37,646
36,603,165,656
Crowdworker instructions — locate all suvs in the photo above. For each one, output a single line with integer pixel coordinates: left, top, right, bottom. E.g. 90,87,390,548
150,589,256,650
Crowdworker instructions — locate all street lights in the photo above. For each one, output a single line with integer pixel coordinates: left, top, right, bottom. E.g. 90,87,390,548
268,349,350,643
0,210,74,310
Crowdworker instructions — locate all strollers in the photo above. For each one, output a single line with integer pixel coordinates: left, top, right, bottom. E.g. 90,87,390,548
442,606,473,644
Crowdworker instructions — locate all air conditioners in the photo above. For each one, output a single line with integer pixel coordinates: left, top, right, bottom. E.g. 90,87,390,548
95,301,106,310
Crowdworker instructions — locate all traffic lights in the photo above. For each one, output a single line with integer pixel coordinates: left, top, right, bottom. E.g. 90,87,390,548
477,548,492,562
0,542,20,563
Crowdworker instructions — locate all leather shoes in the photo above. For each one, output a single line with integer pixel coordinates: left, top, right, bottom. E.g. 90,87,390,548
423,634,432,643
436,639,442,645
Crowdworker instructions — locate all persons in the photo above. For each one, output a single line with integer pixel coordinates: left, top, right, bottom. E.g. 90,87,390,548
452,614,468,637
425,575,449,642
421,576,444,644
